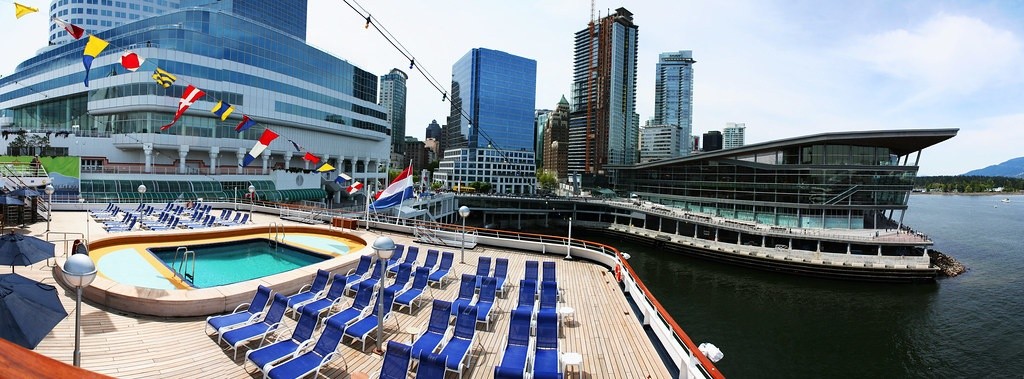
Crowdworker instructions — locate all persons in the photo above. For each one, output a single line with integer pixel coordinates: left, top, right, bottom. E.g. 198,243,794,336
29,154,42,177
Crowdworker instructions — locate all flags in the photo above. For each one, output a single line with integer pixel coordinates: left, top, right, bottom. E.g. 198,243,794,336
300,152,321,163
159,84,205,131
117,50,146,72
334,173,352,184
367,165,414,211
82,35,109,88
52,17,84,40
14,2,38,20
345,180,363,195
288,139,304,152
238,129,279,168
232,114,257,134
151,66,176,89
211,100,233,120
312,162,336,174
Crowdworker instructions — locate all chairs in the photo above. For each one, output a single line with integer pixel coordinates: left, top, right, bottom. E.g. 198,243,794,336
204,242,563,379
91,202,249,233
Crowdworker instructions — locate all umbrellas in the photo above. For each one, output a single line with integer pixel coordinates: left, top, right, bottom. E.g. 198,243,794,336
6,187,39,204
0,272,67,350
0,196,27,223
0,229,56,274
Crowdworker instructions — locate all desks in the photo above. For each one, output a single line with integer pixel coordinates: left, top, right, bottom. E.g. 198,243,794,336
351,371,369,379
560,307,575,327
405,327,420,344
562,353,583,379
